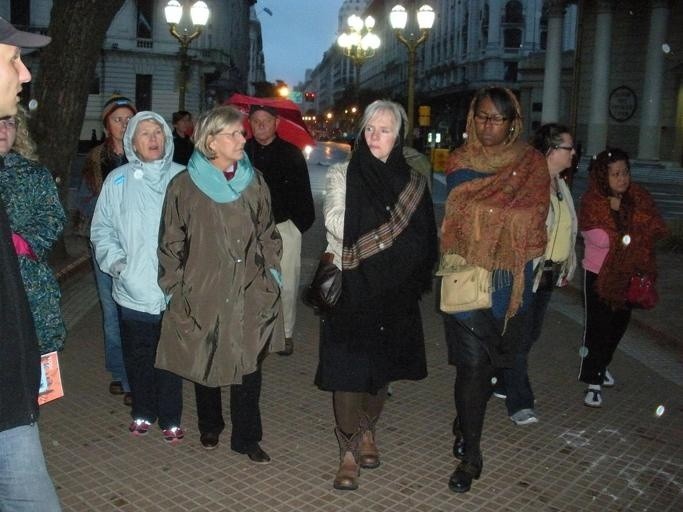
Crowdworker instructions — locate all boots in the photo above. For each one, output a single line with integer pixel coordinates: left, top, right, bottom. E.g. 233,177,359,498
448,436,481,493
360,413,379,469
452,415,466,458
333,430,361,489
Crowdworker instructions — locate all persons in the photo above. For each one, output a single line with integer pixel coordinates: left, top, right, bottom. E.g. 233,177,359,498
81,96,316,464
313,83,665,493
0,17,72,512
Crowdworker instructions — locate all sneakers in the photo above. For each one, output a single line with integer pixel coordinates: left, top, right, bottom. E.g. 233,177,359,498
125,392,132,405
110,381,121,395
602,367,613,387
276,338,292,356
162,427,183,443
128,414,151,435
508,408,538,426
584,385,601,407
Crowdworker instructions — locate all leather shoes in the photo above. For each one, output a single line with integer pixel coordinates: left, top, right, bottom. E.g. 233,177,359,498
200,432,217,447
230,441,270,464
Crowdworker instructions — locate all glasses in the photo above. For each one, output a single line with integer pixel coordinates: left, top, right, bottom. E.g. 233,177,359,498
600,149,624,164
472,113,505,125
214,129,247,139
555,146,572,151
0,120,15,128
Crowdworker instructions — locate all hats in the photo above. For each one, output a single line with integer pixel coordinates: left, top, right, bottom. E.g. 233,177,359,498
0,17,51,53
101,93,137,125
248,104,276,118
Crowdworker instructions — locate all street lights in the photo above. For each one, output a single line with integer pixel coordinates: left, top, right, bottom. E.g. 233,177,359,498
336,13,377,137
161,0,211,137
387,2,437,160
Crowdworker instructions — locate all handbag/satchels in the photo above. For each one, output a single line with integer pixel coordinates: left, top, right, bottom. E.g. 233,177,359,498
435,264,491,314
301,261,341,311
630,275,658,309
